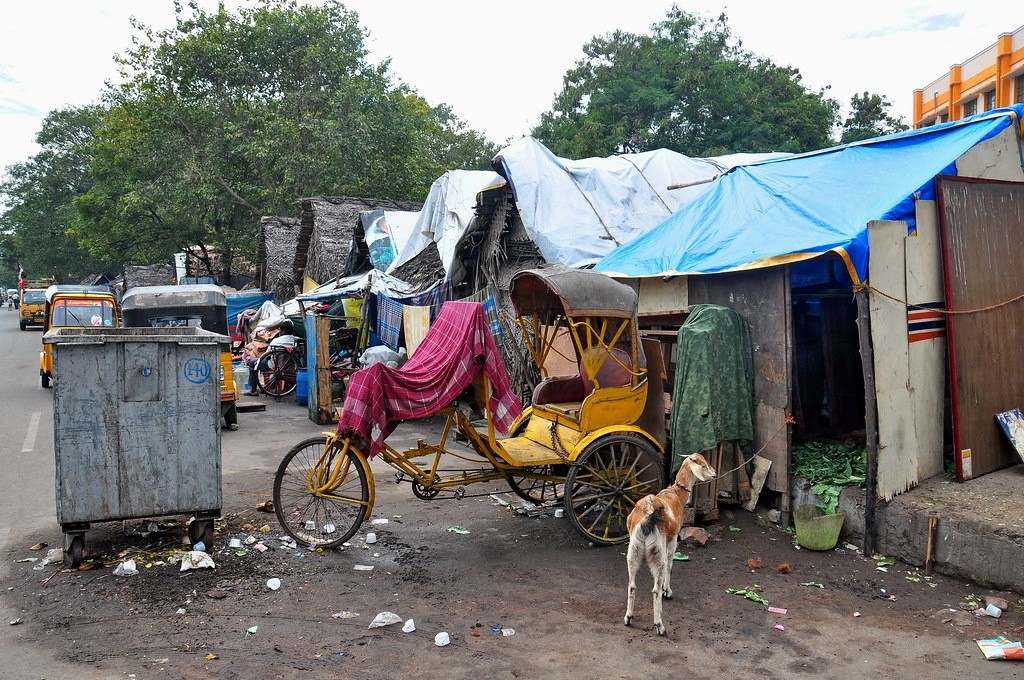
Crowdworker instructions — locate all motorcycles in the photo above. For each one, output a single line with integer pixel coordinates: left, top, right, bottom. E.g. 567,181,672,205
120,284,239,431
38,285,121,388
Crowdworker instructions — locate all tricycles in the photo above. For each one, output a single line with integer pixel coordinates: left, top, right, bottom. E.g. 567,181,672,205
271,268,668,550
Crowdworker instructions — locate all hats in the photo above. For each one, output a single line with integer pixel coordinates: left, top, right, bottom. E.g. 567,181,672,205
277,319,294,328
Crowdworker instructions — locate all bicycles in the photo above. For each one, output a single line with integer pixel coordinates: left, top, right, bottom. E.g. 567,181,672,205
254,332,361,402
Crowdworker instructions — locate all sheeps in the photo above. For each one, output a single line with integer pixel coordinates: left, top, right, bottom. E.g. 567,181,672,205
623,453,718,637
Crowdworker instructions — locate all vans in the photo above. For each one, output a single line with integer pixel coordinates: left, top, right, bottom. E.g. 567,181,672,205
7,289,19,300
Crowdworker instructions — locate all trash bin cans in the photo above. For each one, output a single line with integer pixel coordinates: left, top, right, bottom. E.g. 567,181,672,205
42,322,230,565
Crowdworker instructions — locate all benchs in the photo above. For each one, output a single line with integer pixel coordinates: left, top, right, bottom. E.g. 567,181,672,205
531,345,632,433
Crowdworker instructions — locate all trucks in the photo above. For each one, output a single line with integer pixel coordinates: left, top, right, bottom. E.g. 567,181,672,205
19,289,48,331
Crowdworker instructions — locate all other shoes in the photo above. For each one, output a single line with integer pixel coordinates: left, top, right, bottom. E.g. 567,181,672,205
243,390,259,396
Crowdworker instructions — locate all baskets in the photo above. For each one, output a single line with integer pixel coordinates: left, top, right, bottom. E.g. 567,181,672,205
336,335,357,350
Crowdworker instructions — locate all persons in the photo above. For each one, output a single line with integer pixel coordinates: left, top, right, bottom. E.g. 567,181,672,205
7,292,19,311
243,321,305,396
